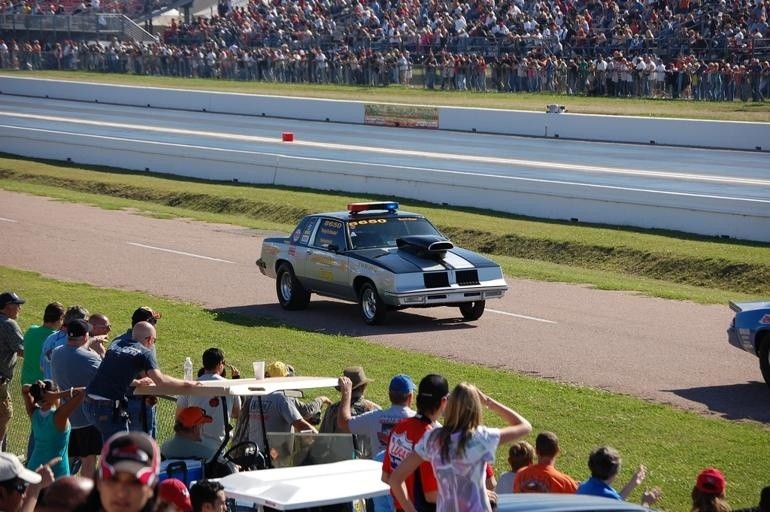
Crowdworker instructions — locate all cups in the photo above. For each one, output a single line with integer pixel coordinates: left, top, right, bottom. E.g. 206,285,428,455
253,361,264,380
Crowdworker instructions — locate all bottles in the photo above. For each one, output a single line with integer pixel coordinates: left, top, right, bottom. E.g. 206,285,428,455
183,357,193,381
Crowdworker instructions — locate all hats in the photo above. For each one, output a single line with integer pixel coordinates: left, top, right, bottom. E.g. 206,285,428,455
68,319,94,337
390,374,416,394
0,292,25,304
697,468,725,494
175,407,213,429
335,367,376,391
99,431,161,487
265,361,294,377
0,452,42,484
132,306,161,322
418,375,451,400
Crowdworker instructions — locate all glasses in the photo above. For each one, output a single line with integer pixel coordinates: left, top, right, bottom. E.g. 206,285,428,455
97,324,110,329
145,337,156,343
193,408,206,426
0,482,26,493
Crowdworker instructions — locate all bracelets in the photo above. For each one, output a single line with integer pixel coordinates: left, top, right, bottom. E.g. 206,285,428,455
319,397,324,402
486,395,491,407
70,386,74,398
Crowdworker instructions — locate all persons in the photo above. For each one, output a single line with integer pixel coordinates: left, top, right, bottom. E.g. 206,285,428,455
575,445,664,509
49,319,102,481
0,450,63,511
231,365,335,462
83,321,203,441
176,345,242,456
108,305,159,441
495,439,537,493
88,313,111,361
388,381,533,511
188,480,228,511
19,379,88,480
689,467,734,511
484,460,498,506
43,475,105,512
309,364,383,462
0,0,770,107
380,373,451,511
161,406,236,472
20,301,66,470
513,430,582,493
40,305,90,382
248,360,320,469
338,372,419,462
0,291,26,452
92,430,159,511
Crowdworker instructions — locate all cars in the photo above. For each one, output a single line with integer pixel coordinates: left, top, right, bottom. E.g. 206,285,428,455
727,300,769,387
255,201,509,325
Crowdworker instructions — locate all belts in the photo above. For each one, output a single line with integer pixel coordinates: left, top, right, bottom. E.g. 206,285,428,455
86,396,113,408
